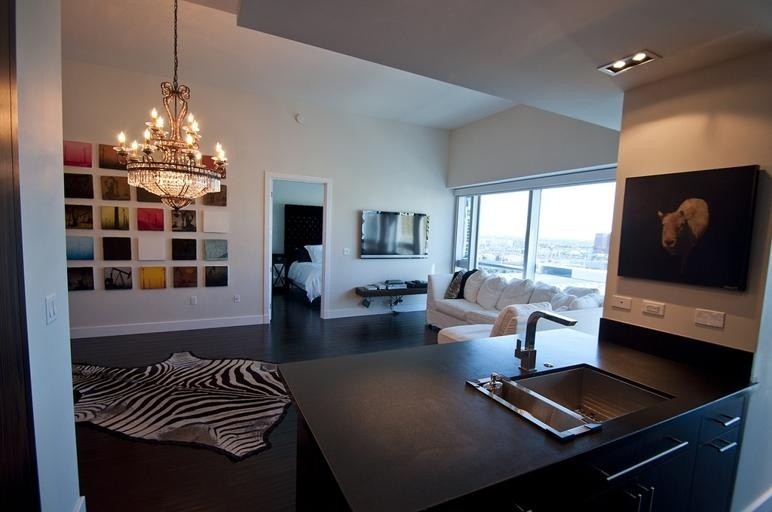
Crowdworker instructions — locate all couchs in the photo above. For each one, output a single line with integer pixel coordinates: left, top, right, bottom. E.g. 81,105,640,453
417,268,605,348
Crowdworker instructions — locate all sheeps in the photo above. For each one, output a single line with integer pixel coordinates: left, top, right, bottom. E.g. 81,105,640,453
657,197,710,256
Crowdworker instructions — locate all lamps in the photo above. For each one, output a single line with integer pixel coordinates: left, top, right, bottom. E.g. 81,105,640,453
114,1,224,209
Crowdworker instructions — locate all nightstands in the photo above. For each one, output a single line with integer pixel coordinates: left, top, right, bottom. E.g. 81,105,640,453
272,253,286,295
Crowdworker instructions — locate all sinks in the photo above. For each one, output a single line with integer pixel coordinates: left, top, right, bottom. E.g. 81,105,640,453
479,380,585,432
518,368,668,422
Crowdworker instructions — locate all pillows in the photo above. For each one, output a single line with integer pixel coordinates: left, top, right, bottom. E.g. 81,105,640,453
294,247,312,264
443,269,577,310
304,244,323,264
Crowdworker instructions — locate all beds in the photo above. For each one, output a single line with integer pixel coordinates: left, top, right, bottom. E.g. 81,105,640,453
284,204,323,306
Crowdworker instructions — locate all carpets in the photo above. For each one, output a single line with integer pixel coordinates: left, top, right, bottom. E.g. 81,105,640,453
71,352,291,462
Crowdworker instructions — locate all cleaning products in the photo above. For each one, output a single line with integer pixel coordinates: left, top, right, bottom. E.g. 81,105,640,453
484,372,502,396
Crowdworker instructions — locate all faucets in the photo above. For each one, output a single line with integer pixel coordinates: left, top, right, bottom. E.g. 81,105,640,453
514,310,577,370
